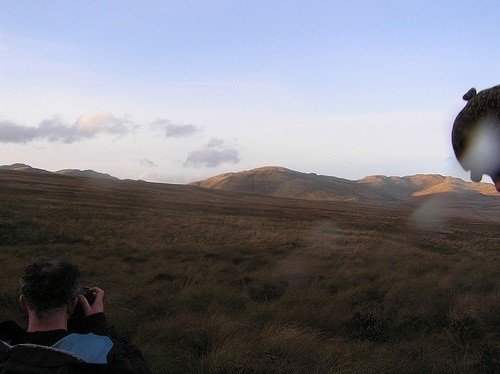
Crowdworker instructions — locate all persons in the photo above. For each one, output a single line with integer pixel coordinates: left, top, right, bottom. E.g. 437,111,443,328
0,256,154,374
451,83,500,192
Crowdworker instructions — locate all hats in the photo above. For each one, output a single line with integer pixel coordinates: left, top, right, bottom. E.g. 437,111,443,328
451,84,500,182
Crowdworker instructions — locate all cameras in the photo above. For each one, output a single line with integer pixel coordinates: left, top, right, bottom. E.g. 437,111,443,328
77,288,97,308
